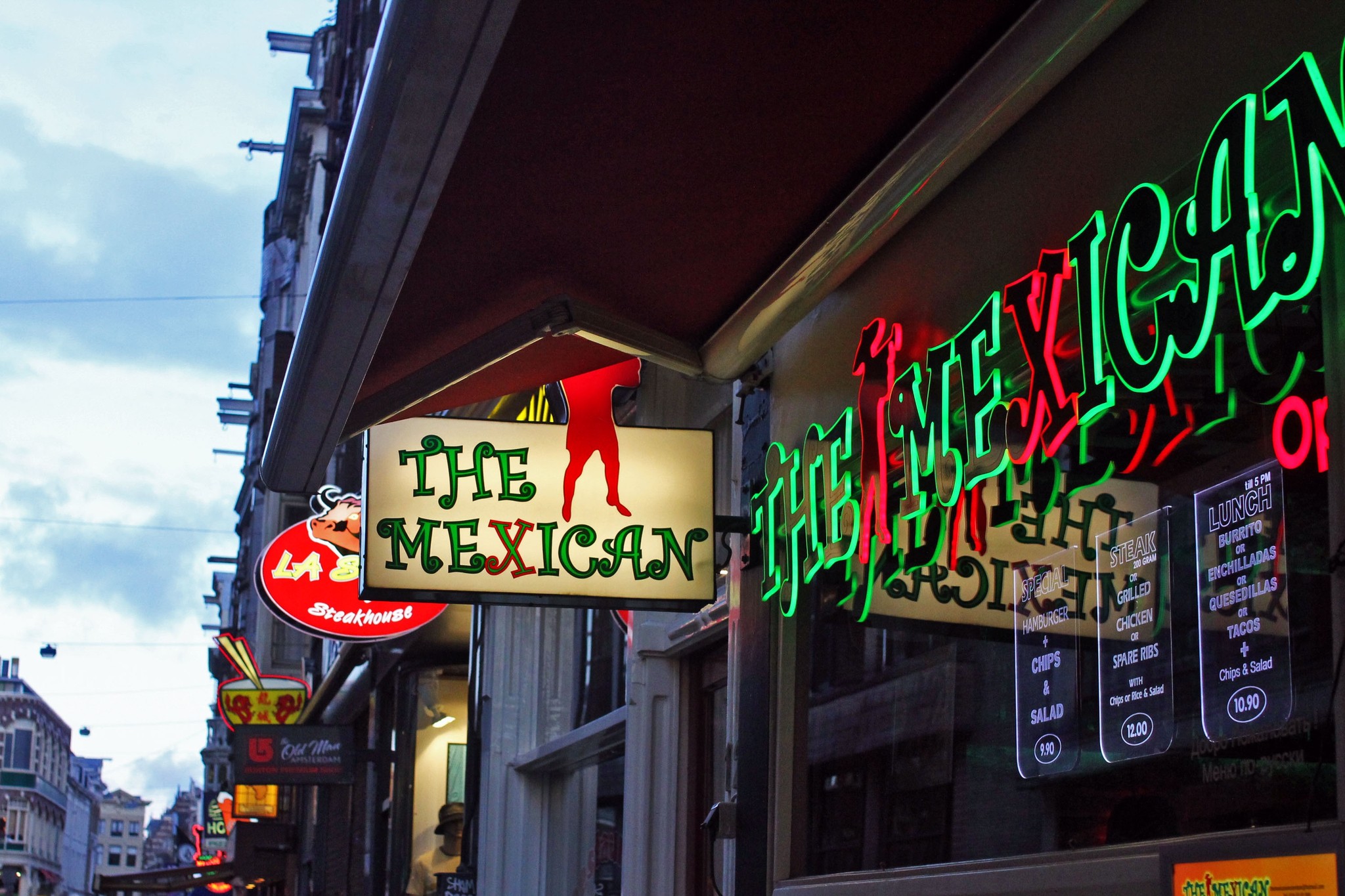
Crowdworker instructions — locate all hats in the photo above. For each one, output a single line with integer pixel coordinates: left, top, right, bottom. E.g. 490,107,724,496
434,802,466,835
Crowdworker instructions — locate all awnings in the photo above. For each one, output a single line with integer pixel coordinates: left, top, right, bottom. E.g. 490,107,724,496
98,857,261,891
40,869,64,885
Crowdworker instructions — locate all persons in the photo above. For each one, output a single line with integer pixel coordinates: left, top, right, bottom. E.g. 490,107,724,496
406,802,466,896
